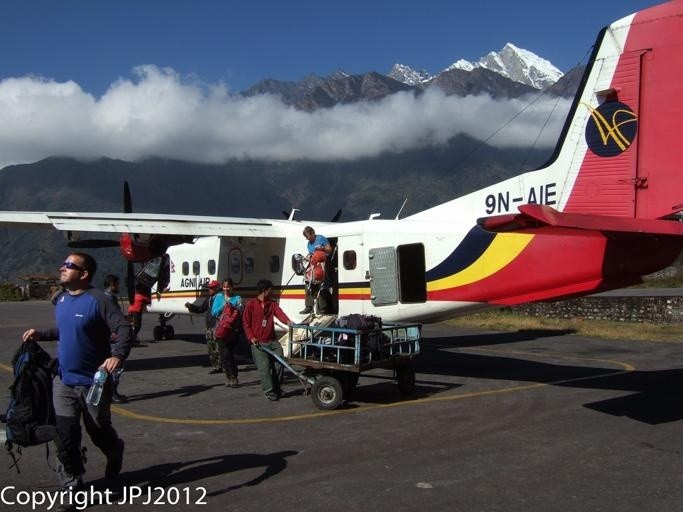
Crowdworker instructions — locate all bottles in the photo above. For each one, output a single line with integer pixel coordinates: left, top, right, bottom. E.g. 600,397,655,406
86,366,108,406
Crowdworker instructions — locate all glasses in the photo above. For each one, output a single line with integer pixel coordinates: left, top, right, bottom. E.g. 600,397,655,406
62,262,84,271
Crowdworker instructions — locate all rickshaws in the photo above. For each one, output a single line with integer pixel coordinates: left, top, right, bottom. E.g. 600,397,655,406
251,312,421,411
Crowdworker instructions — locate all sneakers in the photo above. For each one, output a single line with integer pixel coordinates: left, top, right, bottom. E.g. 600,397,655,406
265,390,279,401
275,391,287,398
209,368,238,388
300,306,313,314
55,486,82,512
105,438,124,481
320,306,328,314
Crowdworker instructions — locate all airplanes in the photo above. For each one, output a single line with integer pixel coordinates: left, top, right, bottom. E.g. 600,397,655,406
2,1,681,338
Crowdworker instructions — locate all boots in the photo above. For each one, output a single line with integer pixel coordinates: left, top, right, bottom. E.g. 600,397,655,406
110,382,127,404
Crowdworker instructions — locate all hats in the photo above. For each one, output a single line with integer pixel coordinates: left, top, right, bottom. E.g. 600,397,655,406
207,281,221,288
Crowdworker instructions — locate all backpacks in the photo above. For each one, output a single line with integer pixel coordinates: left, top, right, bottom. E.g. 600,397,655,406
0,338,59,473
303,314,390,365
207,302,245,339
303,248,327,290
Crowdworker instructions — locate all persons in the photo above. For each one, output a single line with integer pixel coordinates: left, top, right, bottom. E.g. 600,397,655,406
297,226,334,316
21,252,136,512
185,280,223,374
102,273,128,403
211,277,244,388
125,285,151,345
242,280,296,401
